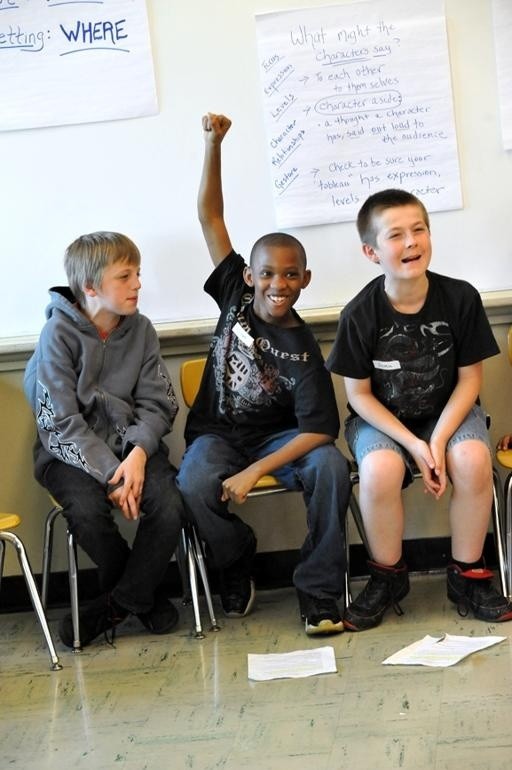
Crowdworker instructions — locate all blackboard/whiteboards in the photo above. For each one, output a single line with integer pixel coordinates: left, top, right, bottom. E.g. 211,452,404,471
0,0,512,372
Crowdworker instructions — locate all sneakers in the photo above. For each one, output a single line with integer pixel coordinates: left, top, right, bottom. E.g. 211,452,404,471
295,585,344,635
218,523,256,618
136,596,180,634
447,554,512,622
344,558,410,631
59,592,129,649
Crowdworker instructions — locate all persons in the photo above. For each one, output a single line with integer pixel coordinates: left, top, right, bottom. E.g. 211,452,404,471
172,109,353,636
20,230,188,650
494,429,512,452
322,186,512,633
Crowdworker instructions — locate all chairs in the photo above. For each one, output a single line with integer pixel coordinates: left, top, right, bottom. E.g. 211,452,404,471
40,491,219,653
341,461,510,614
181,357,357,643
1,512,64,670
498,442,510,571
